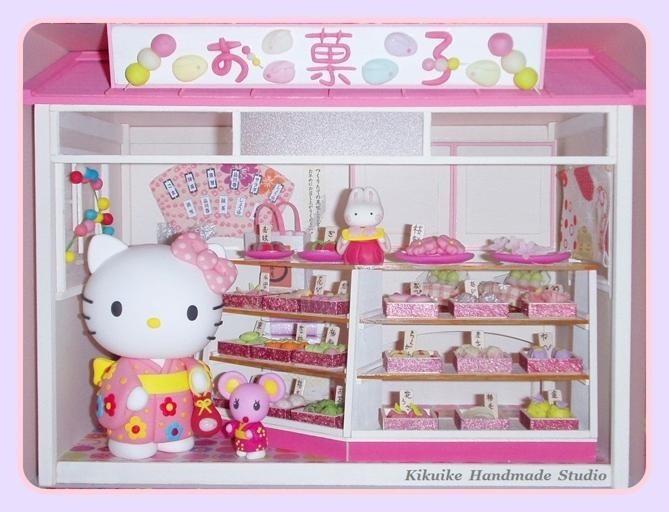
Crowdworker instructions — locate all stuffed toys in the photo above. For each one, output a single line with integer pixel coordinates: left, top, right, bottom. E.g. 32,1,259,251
82,234,237,460
